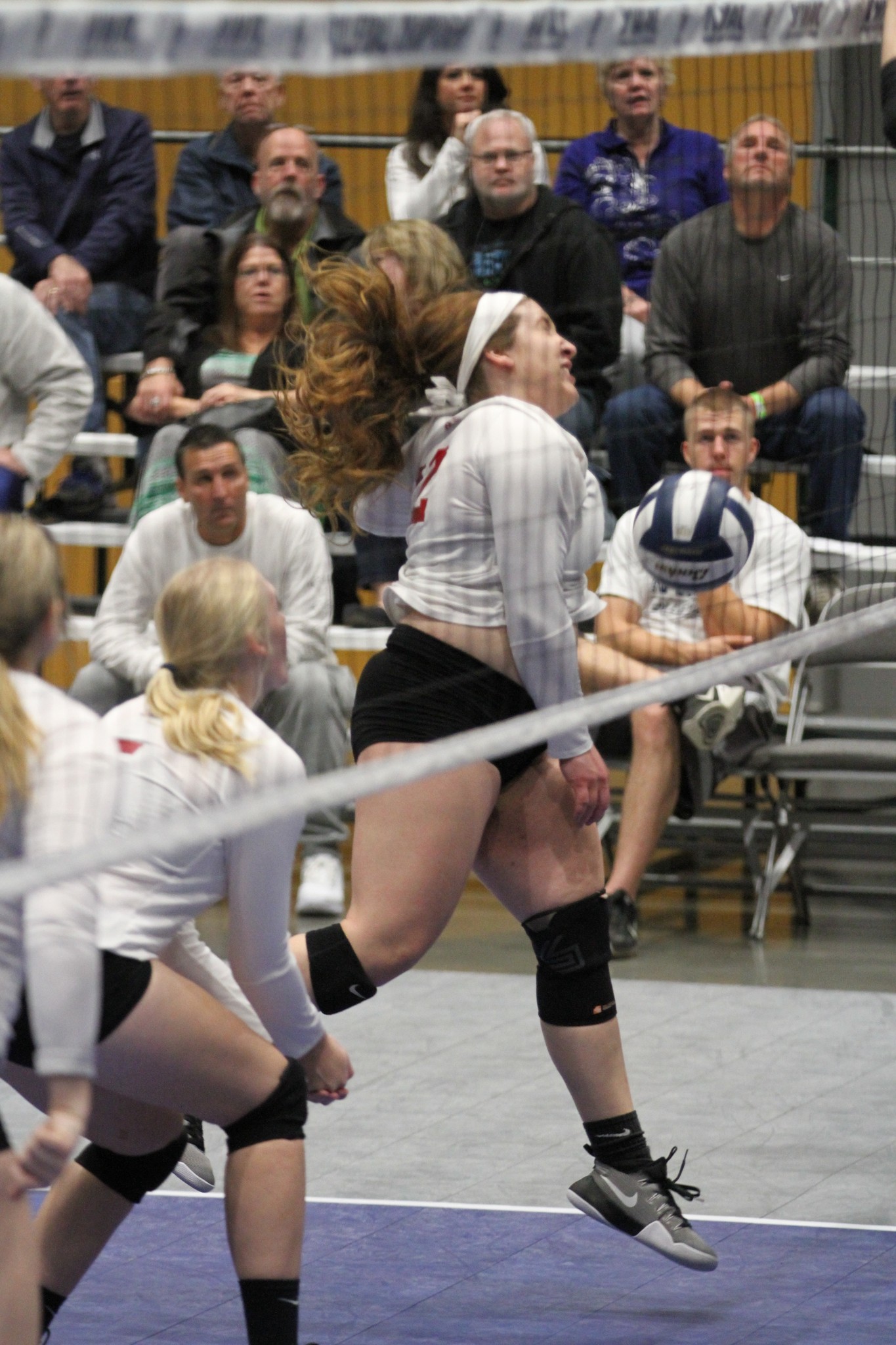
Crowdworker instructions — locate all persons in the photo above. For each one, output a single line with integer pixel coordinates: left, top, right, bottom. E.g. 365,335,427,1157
605,113,868,544
876,2,896,146
577,392,814,960
0,516,121,1345
1,63,730,627
70,423,351,920
39,556,356,1345
173,218,716,1272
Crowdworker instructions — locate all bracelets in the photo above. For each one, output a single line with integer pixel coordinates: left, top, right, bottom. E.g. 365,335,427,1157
750,392,767,420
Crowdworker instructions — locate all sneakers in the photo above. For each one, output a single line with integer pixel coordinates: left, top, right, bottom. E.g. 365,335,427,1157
566,1143,718,1272
172,1114,215,1193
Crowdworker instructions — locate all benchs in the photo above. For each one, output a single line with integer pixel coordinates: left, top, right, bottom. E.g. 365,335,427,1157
32,342,896,661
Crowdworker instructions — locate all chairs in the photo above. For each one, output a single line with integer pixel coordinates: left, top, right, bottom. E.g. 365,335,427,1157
616,581,896,945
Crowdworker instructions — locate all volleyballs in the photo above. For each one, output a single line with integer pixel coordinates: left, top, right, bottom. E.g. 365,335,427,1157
632,469,754,591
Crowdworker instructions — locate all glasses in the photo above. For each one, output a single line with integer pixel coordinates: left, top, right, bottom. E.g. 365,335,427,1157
471,148,532,164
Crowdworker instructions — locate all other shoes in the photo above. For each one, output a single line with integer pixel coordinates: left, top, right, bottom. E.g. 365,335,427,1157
608,888,641,959
28,475,115,524
684,669,777,753
296,852,346,917
